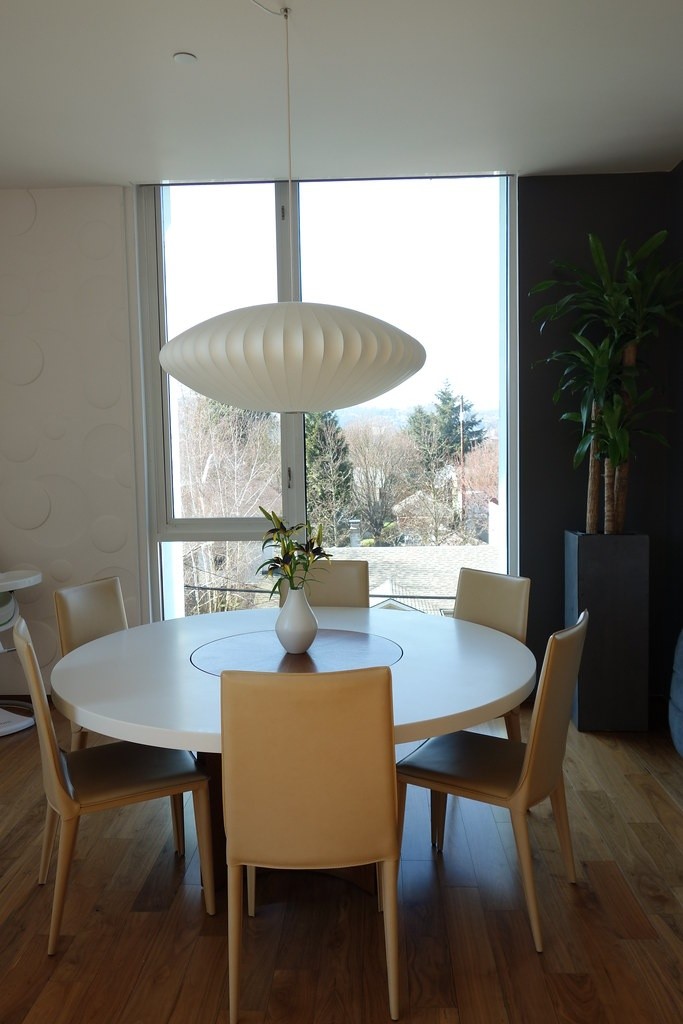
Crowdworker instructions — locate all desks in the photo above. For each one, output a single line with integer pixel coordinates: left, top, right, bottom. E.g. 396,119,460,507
50,606,536,748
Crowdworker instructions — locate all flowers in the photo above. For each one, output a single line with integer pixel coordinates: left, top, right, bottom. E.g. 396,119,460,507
255,506,333,602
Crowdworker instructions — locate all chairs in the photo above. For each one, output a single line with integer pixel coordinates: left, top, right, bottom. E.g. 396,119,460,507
219,667,400,1024
53,576,131,752
395,609,590,951
452,567,531,741
13,616,217,954
279,560,370,607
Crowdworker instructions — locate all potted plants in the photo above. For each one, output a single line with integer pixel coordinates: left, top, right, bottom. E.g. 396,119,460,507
529,230,683,733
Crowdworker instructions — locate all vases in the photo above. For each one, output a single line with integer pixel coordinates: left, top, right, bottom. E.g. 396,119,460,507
275,587,319,654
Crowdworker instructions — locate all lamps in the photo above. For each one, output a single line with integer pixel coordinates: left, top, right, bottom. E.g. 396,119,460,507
159,0,427,414
